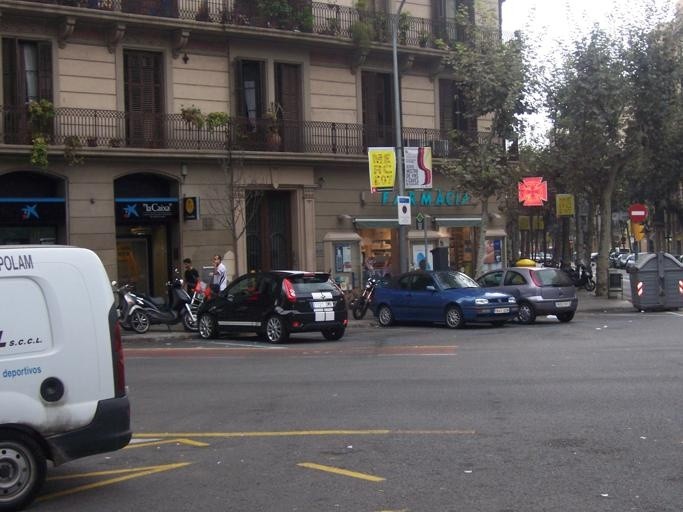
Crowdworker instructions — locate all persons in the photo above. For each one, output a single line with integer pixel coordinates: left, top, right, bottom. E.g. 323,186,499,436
209,254,227,292
180,257,199,306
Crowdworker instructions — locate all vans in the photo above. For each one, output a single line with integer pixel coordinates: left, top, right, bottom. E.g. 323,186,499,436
0,244,133,512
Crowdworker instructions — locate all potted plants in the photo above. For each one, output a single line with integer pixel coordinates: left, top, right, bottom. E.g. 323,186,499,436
264,102,284,151
416,29,432,47
256,0,294,28
63,136,86,169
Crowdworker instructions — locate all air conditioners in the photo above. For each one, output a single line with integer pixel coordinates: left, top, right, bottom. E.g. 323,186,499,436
403,138,448,157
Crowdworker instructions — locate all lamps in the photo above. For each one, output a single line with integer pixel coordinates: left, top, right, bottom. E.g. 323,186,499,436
338,215,351,221
181,162,188,180
182,52,189,64
490,213,501,219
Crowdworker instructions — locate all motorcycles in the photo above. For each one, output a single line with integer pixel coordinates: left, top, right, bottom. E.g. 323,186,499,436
111,268,218,330
351,276,381,320
566,264,595,292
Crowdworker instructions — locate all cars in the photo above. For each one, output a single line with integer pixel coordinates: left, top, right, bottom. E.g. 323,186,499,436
591,245,649,268
196,269,347,342
371,269,518,330
517,247,555,263
474,267,579,324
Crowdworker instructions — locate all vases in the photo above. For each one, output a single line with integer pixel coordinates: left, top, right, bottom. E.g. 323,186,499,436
87,136,123,147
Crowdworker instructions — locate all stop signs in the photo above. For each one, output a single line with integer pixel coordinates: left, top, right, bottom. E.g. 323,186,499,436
628,204,648,223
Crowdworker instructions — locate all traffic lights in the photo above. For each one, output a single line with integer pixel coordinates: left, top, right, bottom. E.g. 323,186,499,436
632,224,646,243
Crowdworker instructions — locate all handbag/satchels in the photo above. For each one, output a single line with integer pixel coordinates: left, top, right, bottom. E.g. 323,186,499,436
209,284,220,293
